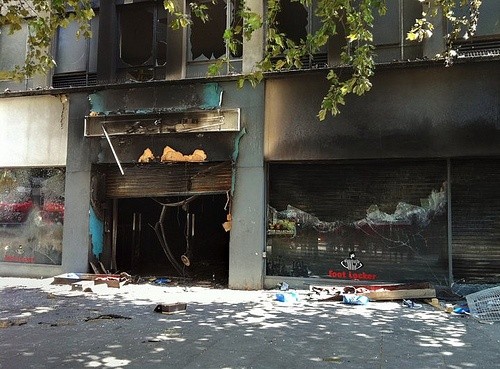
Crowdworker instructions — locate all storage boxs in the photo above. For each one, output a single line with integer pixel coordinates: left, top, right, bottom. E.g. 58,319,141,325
464,285,500,321
